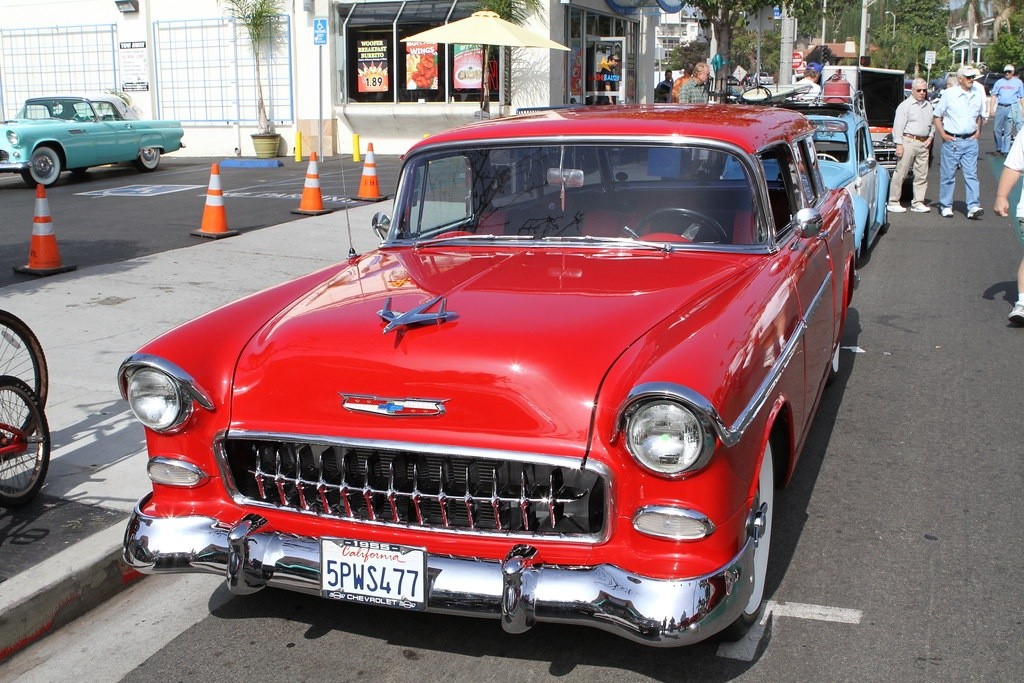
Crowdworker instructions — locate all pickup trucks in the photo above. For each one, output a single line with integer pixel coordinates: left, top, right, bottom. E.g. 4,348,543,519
749,71,775,86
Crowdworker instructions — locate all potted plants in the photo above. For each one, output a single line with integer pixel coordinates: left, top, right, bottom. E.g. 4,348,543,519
216,0,287,159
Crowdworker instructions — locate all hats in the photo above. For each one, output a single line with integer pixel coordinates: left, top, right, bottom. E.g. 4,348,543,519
806,62,821,73
957,67,977,76
1003,64,1014,71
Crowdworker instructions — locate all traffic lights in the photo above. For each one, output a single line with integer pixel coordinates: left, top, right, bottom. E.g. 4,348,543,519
860,56,871,66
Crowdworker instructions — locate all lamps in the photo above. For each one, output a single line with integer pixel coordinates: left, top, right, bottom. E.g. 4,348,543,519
114,0,139,12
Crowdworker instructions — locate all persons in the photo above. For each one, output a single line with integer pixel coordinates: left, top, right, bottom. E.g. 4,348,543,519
598,54,622,104
886,77,936,212
656,70,675,103
671,63,694,103
993,125,1024,325
933,66,985,219
679,63,711,104
958,65,1024,169
792,62,825,101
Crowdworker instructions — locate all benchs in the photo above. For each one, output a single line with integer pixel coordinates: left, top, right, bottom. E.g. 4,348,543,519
569,187,789,231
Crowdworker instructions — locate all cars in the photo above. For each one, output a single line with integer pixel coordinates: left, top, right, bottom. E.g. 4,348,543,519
719,102,890,265
903,71,1006,103
116,100,862,650
0,94,186,189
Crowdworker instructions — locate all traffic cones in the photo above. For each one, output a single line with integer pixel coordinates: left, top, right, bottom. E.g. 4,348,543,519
12,184,78,277
351,142,389,203
189,162,240,239
290,151,334,216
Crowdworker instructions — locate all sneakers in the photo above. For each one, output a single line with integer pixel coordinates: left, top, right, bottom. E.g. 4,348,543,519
910,204,930,212
1008,303,1024,325
940,206,953,217
967,206,983,219
888,204,906,212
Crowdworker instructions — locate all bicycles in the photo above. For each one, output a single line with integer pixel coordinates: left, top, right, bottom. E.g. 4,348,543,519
0,308,52,509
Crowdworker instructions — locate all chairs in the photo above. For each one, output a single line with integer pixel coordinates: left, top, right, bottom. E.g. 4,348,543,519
620,209,756,244
475,210,620,237
59,105,78,119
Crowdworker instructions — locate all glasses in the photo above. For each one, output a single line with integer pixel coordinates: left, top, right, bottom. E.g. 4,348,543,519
963,75,976,79
1005,70,1012,73
913,88,927,93
703,72,709,77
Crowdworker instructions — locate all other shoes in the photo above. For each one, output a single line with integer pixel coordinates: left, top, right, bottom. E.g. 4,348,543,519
994,150,1001,156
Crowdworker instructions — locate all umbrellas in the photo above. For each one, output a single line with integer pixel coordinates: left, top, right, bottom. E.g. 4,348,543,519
399,12,572,108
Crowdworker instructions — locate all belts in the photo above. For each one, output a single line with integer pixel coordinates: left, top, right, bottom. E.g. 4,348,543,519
998,103,1011,108
903,133,928,141
944,131,976,139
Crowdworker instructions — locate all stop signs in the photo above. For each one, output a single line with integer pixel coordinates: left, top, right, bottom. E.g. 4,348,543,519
791,53,802,69
